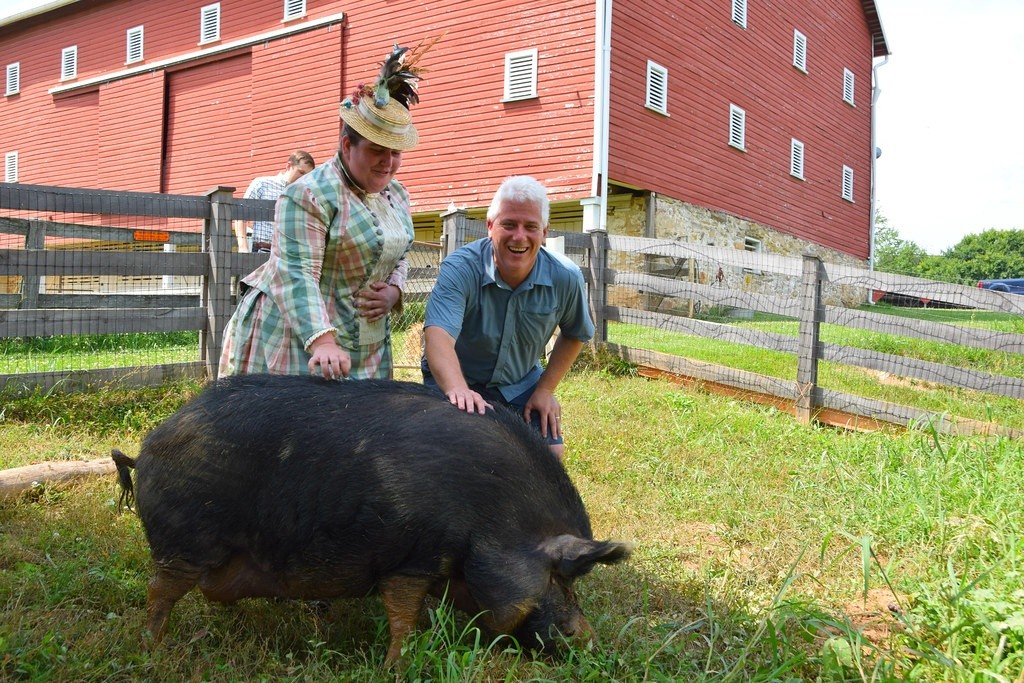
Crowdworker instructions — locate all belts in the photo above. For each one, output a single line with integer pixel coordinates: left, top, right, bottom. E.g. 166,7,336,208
254,242,271,248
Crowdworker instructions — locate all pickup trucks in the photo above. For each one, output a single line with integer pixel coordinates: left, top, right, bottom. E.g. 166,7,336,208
978,278,1023,294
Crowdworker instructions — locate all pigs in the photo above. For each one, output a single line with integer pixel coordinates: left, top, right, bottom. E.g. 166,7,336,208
109,369,633,677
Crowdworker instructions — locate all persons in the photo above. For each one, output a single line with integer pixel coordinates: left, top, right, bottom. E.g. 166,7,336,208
420,173,594,461
219,102,414,382
235,150,315,253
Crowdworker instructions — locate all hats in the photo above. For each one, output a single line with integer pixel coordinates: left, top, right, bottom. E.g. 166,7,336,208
338,29,450,151
